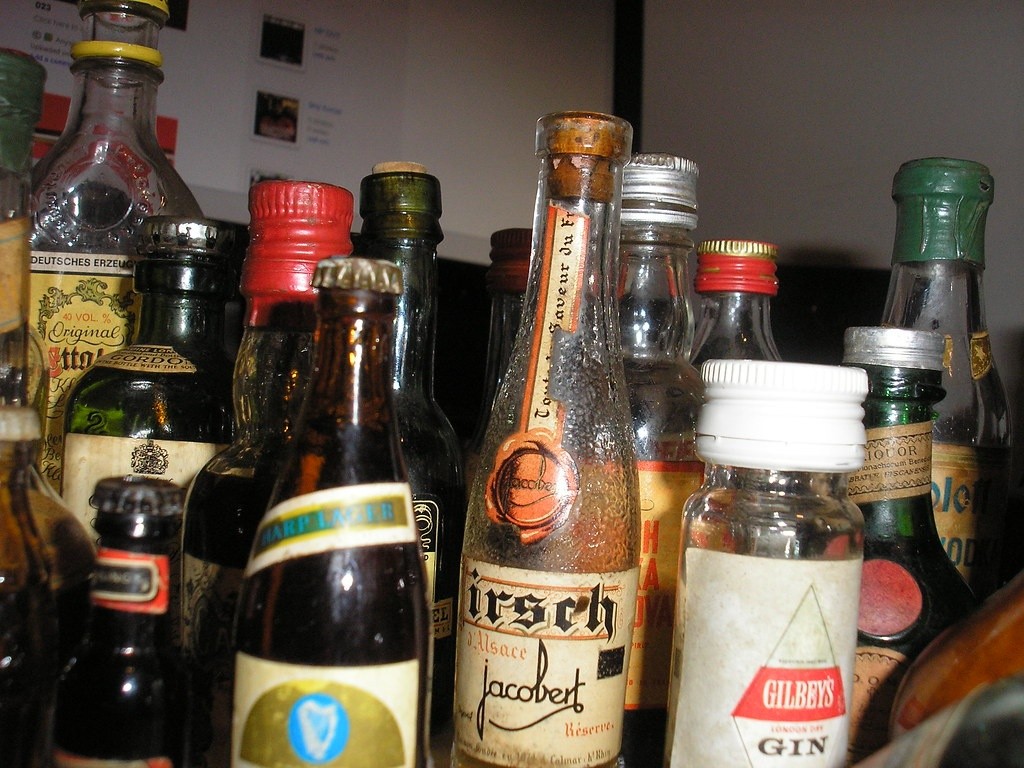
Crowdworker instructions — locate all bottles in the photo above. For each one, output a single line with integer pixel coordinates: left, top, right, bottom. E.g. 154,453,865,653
0,0,1024,768
